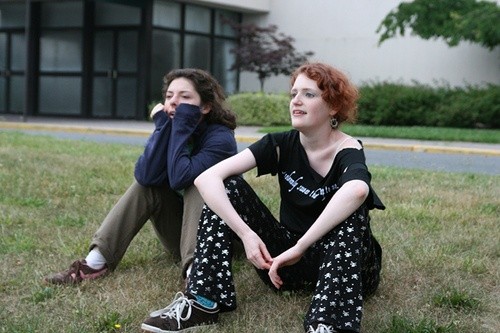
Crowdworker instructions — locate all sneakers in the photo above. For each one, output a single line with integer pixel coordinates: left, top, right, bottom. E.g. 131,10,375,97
308,324,335,333
141,292,221,333
43,258,109,289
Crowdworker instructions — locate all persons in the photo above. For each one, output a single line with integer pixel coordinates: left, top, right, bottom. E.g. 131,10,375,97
141,63,386,333
43,68,238,294
